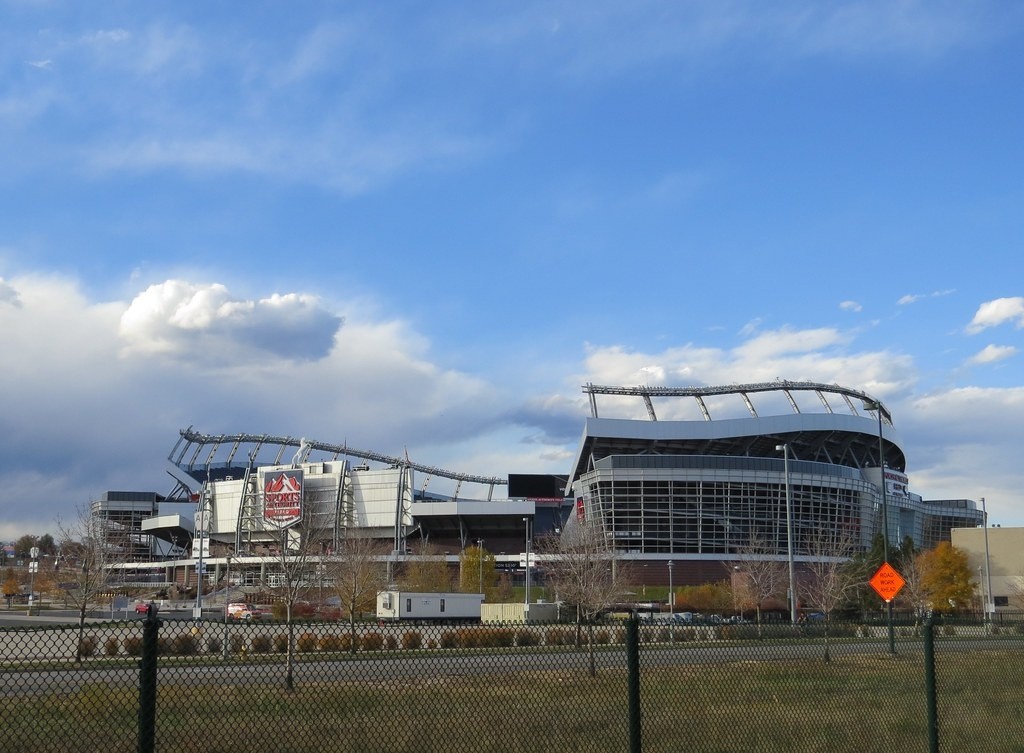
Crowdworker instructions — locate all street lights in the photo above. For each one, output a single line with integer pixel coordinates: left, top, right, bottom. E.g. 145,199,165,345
979,497,993,625
523,517,529,604
776,443,797,625
196,489,210,619
667,559,676,647
477,541,482,594
864,401,900,656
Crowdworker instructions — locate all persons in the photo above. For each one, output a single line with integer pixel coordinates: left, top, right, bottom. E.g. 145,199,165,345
795,612,810,627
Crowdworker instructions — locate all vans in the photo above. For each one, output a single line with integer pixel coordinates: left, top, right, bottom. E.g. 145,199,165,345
226,603,273,623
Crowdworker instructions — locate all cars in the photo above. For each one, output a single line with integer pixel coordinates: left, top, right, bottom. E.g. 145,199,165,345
136,603,153,615
